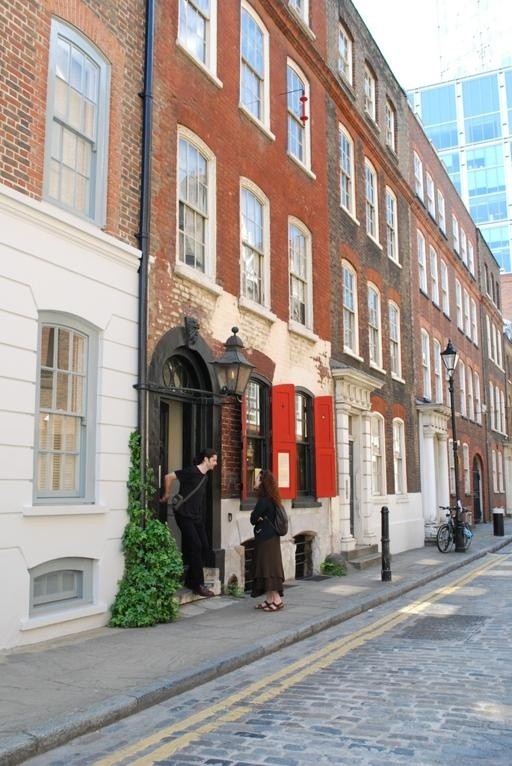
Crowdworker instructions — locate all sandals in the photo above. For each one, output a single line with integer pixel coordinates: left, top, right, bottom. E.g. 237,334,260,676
254,600,268,610
264,601,285,612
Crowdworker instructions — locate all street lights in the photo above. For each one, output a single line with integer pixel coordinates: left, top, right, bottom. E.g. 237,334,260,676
440,337,465,553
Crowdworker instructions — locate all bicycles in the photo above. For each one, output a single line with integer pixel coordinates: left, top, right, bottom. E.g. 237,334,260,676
436,506,470,553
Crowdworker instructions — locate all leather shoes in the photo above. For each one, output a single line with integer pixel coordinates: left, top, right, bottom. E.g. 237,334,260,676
192,585,215,597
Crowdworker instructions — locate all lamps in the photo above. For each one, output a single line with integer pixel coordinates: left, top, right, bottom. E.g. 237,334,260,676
210,324,257,400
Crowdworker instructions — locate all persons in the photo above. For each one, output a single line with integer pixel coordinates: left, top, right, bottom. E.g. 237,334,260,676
249,469,285,612
160,450,218,599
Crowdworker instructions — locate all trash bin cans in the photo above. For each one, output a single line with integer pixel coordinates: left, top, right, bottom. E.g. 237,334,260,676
492,508,504,536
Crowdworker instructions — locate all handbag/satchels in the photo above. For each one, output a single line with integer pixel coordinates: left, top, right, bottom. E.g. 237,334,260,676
172,495,183,509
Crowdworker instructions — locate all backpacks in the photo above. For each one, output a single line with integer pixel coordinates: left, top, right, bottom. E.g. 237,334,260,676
273,501,288,535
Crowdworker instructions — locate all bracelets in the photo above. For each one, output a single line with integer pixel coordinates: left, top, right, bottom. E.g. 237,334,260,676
164,495,169,501
